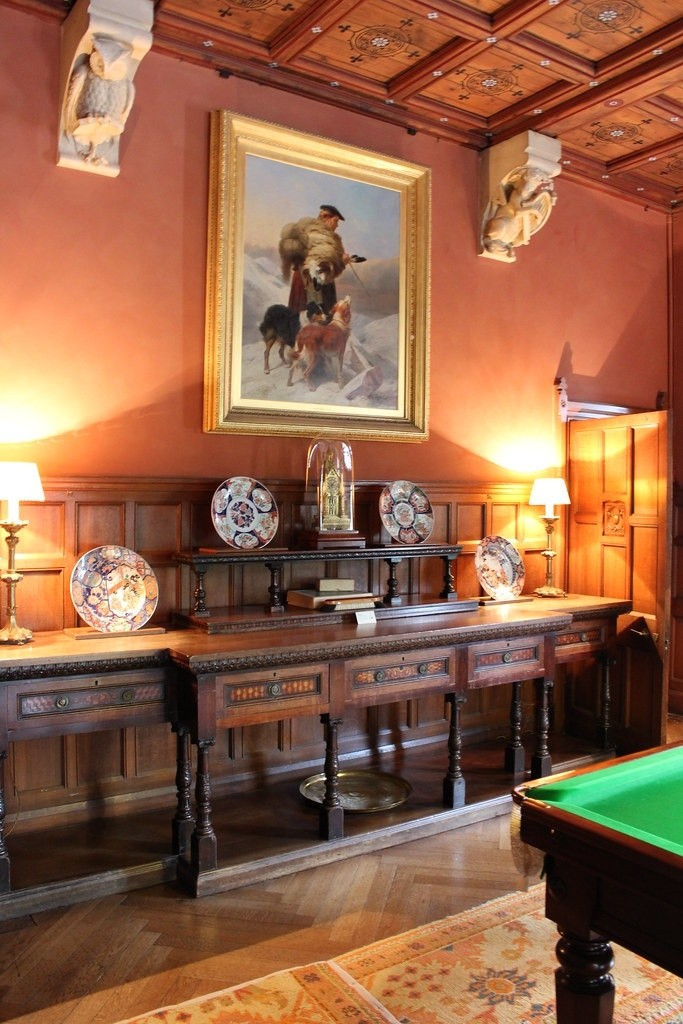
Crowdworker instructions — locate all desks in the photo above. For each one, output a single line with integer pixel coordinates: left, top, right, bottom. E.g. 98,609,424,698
171,536,463,618
0,594,632,897
511,739,683,1024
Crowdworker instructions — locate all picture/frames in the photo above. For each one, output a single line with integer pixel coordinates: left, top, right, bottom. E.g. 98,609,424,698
202,107,433,442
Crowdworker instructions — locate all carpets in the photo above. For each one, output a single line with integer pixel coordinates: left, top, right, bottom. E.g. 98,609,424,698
113,884,683,1024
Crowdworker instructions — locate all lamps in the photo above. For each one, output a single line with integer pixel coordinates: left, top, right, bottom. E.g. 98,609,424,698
0,460,46,647
528,477,571,597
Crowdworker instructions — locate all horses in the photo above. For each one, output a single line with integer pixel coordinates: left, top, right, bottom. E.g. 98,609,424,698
482,168,552,257
